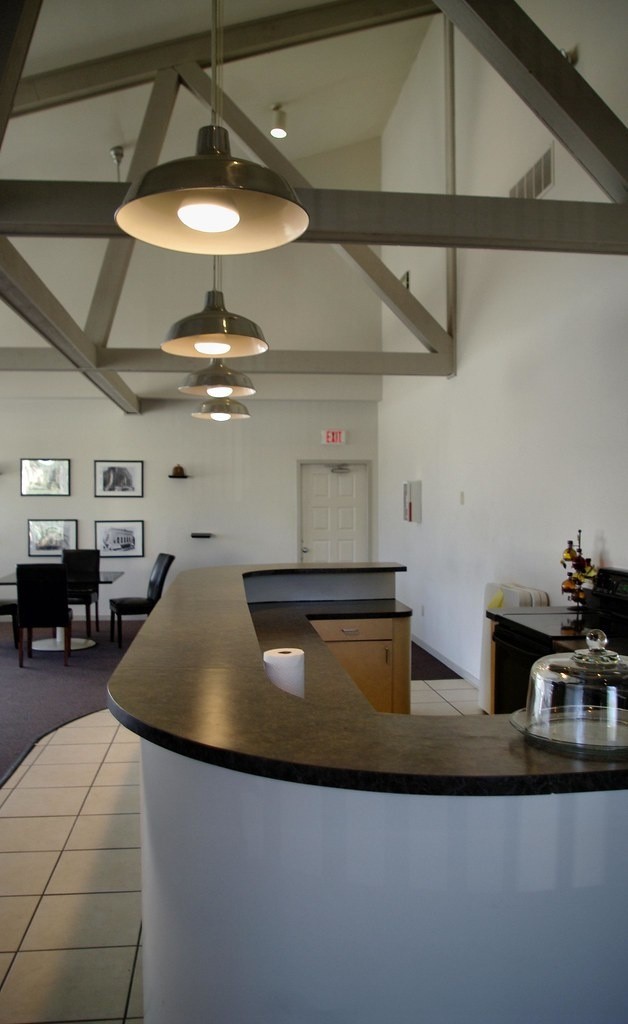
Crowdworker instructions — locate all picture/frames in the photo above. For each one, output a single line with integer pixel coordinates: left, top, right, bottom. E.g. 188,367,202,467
93,460,144,498
26,519,78,556
93,520,145,558
19,458,71,497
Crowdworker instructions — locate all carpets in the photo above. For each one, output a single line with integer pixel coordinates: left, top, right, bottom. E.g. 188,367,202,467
0,619,149,788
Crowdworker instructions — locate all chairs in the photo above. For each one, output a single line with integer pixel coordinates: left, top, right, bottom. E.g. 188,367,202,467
15,564,74,668
0,599,18,650
109,553,176,647
61,549,101,636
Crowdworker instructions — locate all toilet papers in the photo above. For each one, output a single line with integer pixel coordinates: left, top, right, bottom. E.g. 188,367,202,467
262,647,306,701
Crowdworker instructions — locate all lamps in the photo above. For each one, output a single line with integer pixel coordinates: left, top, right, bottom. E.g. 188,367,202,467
159,257,269,357
190,400,252,422
176,358,257,399
118,0,312,254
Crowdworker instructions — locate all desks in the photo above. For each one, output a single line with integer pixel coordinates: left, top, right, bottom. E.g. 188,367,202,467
0,570,124,652
